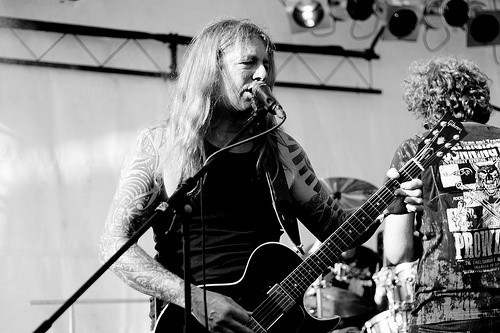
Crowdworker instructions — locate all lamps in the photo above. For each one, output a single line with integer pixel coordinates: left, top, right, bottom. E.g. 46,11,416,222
280,0,500,63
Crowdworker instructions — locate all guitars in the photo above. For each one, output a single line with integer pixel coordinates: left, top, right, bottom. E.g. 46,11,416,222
151,108,470,333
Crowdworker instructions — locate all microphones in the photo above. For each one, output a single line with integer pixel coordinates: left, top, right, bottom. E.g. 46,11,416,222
251,80,286,120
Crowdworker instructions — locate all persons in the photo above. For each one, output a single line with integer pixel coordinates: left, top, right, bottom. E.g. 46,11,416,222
99,15,424,333
303,237,381,302
384,53,500,333
